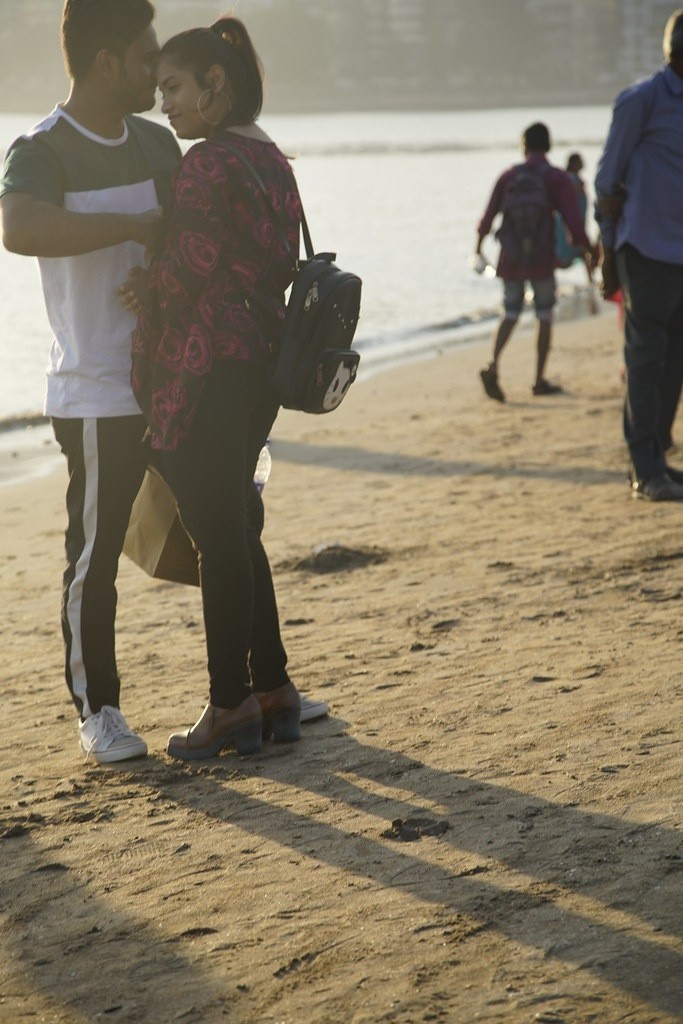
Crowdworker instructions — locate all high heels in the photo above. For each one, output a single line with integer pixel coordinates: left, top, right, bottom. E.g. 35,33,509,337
167,694,263,759
251,682,302,742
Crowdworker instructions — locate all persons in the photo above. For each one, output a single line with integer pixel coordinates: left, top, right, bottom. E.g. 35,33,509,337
477,122,596,404
594,10,683,501
1,1,329,764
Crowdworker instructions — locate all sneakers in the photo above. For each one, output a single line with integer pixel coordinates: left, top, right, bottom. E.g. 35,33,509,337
79,705,147,765
299,694,328,722
630,473,683,502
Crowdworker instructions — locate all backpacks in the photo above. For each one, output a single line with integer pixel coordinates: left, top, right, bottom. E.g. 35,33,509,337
205,138,362,414
494,165,554,262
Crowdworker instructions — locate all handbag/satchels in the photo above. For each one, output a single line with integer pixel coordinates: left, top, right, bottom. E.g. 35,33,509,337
121,465,200,585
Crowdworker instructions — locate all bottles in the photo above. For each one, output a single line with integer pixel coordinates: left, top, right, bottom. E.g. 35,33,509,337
252,438,272,495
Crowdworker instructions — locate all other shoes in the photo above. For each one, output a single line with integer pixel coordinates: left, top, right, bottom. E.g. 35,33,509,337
481,370,504,401
532,382,562,395
